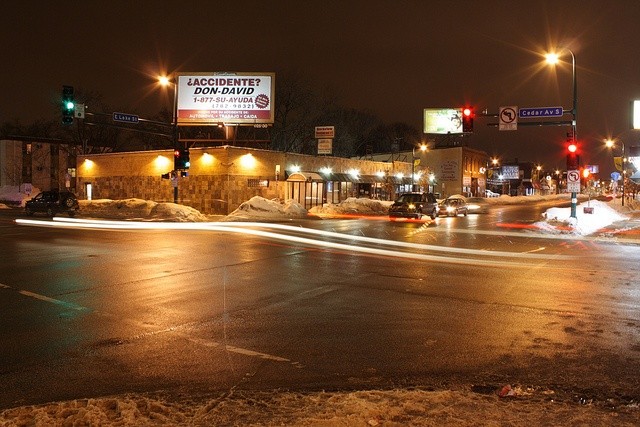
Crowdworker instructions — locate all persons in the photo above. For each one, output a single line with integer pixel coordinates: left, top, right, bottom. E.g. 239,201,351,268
451,117,461,128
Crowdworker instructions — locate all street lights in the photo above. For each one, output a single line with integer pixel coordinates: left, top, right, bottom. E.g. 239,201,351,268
545,43,578,220
607,137,625,206
412,143,426,191
159,77,178,122
485,158,498,198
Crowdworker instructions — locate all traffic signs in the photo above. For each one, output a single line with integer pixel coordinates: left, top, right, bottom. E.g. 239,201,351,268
113,111,139,124
518,106,564,119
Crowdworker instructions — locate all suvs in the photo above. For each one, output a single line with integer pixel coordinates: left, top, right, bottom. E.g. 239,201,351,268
388,190,438,219
24,189,79,217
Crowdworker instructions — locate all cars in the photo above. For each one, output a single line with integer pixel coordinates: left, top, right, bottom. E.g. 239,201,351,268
437,197,468,216
465,197,489,213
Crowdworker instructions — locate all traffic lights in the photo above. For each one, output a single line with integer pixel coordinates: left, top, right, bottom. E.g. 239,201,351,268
62,93,75,125
566,140,578,170
174,146,182,169
462,106,473,132
182,147,190,168
